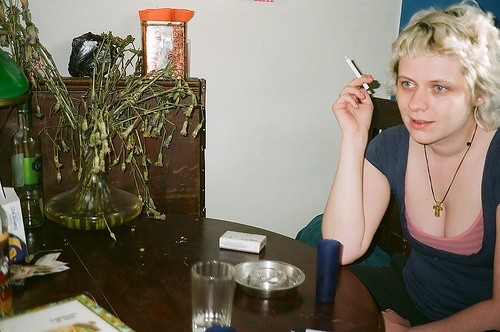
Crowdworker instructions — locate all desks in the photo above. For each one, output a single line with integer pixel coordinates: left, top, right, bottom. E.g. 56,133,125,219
0,213,386,332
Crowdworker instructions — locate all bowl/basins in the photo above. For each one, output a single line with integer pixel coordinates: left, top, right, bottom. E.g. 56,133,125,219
234,260,306,298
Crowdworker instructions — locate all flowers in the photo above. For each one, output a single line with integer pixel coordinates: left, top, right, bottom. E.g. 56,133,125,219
0,0,207,240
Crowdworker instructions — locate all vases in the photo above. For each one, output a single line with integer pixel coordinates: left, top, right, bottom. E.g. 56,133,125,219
44,140,143,230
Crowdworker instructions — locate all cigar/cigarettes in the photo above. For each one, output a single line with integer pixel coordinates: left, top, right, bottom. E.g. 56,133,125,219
344,56,369,90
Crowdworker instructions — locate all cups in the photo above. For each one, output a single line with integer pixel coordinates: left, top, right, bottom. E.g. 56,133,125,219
191,261,236,332
206,327,235,332
316,239,341,304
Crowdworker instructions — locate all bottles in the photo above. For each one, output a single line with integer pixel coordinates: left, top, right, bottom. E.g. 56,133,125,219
10,102,45,229
0,204,13,313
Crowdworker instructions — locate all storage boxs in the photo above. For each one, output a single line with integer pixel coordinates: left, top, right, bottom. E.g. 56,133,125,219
219,231,266,253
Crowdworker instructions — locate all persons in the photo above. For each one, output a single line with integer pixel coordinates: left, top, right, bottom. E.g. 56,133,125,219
322,3,500,332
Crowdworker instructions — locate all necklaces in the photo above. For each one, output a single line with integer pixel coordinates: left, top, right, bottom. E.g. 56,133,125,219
424,124,478,217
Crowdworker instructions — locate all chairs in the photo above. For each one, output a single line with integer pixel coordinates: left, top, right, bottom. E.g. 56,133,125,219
295,98,408,266
